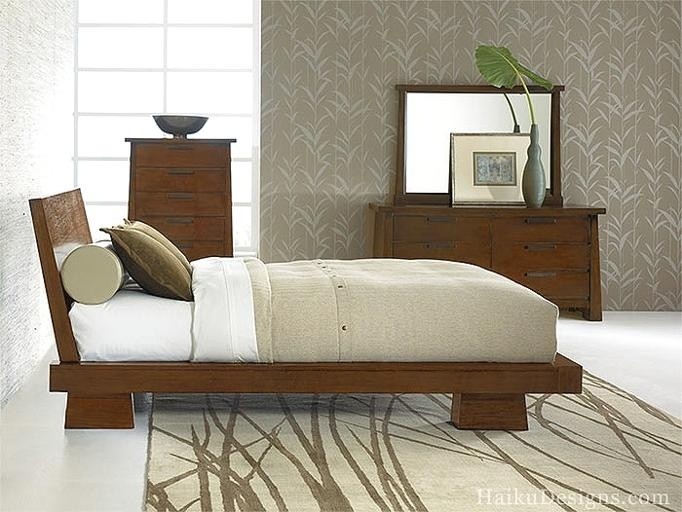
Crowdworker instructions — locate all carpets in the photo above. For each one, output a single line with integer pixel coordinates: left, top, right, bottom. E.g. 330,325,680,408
139,370,682,512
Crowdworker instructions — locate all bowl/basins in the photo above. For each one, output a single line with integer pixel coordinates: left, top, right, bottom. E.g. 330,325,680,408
152,115,210,135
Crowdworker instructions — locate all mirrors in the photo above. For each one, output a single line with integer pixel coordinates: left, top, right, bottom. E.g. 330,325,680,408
394,83,565,206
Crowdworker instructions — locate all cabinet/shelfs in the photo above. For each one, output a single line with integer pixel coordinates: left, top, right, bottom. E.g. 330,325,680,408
122,136,237,260
27,189,582,428
366,199,606,321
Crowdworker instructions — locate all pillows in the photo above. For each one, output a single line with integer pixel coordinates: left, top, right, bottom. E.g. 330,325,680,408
100,225,194,300
57,239,127,305
105,216,193,275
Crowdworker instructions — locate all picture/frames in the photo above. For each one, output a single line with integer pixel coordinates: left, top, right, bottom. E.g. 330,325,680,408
450,132,531,208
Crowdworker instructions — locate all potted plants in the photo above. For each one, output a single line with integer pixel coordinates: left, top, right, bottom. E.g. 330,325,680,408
474,42,554,209
